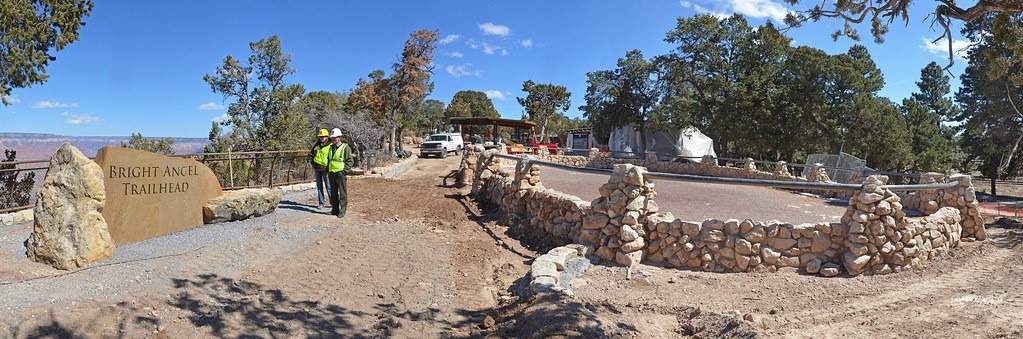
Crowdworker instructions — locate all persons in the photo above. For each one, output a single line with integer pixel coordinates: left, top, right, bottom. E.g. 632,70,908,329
324,128,354,217
310,128,334,209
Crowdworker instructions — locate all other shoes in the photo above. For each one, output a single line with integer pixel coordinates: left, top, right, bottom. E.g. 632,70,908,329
327,212,339,215
317,205,323,209
338,212,344,218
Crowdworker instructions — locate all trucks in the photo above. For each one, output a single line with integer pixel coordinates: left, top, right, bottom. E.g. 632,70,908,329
471,132,563,154
420,132,464,158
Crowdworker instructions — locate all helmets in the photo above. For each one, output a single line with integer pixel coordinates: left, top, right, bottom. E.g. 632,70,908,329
317,129,329,136
329,128,342,137
